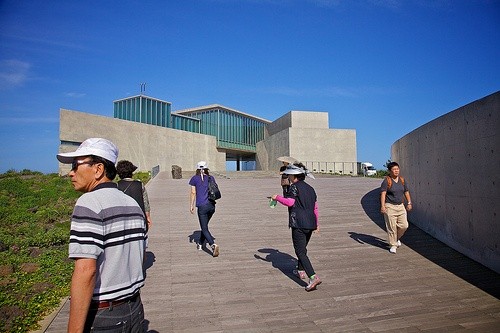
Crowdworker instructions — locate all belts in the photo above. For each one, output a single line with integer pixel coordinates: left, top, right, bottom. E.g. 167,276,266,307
90,291,139,309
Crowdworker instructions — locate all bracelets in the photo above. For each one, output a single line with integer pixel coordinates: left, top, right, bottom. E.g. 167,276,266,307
408,202,412,205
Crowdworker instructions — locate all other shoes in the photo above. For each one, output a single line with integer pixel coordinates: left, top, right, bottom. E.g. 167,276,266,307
397,240,401,246
390,246,397,253
197,243,204,250
212,245,219,256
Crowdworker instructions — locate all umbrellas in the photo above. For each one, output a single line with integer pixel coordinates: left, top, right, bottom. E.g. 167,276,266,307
276,156,299,167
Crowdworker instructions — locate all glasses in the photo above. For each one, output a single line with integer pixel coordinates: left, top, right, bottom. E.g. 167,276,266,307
72,161,98,171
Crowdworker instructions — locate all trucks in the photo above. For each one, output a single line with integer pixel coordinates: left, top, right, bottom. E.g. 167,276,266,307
357,162,377,176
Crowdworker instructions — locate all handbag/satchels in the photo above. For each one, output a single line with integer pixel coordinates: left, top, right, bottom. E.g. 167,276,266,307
208,175,221,200
281,178,290,185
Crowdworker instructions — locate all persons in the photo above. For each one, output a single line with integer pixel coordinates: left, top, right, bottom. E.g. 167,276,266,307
381,162,412,254
280,161,291,198
56,138,150,333
189,161,220,258
272,163,322,291
115,160,152,229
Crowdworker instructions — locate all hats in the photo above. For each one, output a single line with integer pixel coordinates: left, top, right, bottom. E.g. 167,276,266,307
115,160,138,172
197,161,210,169
57,138,118,164
282,165,309,174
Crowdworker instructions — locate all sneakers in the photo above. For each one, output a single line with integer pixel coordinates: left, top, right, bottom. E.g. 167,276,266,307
305,274,322,290
292,269,305,279
